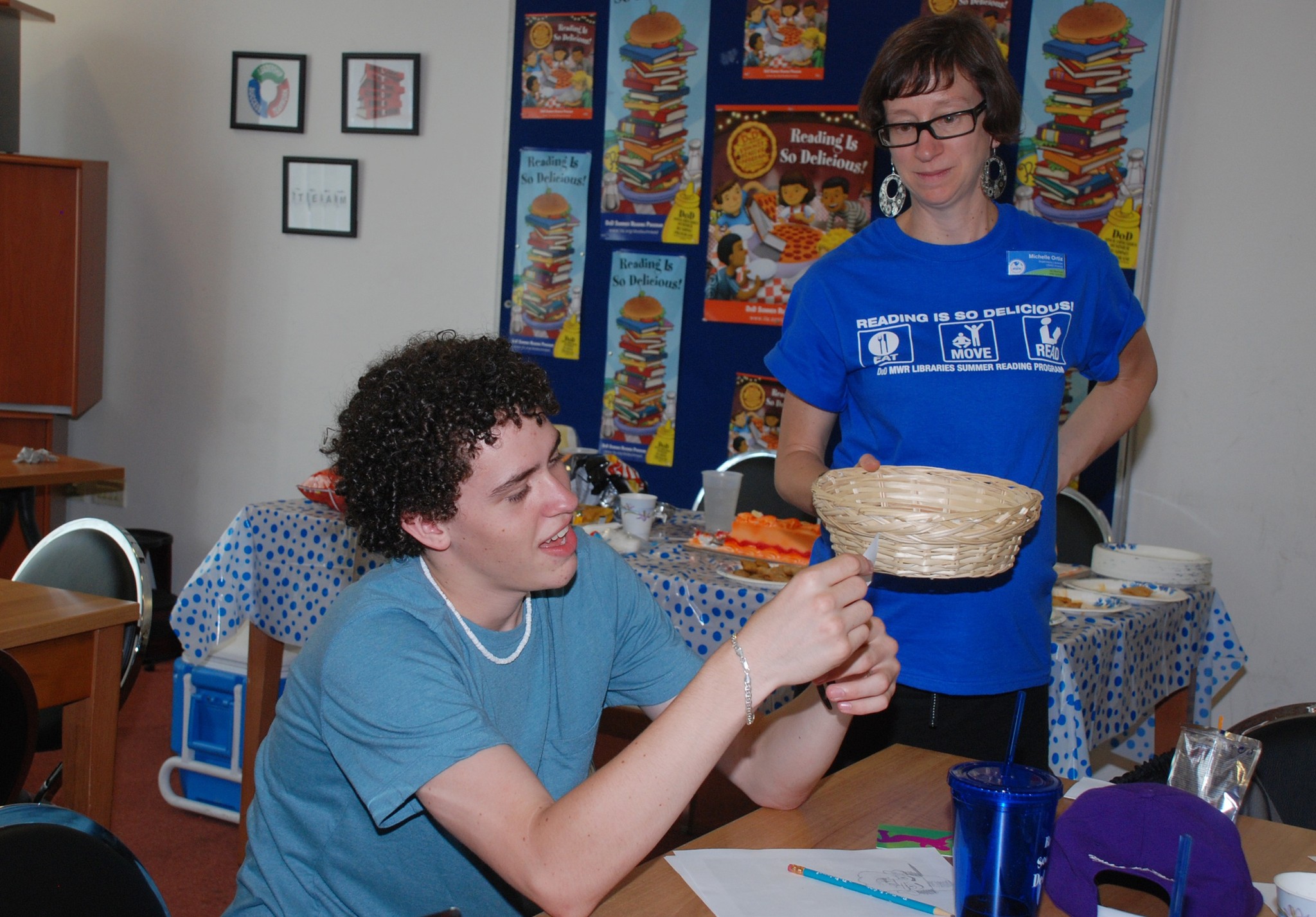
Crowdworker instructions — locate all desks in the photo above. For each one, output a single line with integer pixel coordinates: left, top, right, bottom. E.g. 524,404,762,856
0,579,140,830
528,744,1316,917
0,443,126,551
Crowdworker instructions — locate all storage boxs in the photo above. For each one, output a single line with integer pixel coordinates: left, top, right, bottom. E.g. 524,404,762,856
159,620,301,821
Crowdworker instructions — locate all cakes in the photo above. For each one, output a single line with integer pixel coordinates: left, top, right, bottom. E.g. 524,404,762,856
723,510,822,562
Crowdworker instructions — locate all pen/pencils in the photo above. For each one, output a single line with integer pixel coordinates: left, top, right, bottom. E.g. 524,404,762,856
788,864,955,916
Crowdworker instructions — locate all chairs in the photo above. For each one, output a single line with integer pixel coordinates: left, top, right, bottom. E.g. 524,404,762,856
11,517,153,713
1113,702,1316,830
0,804,172,917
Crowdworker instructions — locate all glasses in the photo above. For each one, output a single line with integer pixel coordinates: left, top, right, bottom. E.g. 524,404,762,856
878,98,988,148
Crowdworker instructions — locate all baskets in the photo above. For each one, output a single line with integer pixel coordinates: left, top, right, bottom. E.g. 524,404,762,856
810,465,1043,578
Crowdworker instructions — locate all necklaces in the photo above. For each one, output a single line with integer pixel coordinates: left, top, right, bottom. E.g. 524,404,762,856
418,556,531,665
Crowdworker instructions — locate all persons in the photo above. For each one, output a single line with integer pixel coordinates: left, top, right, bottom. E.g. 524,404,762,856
223,330,901,917
765,14,1159,767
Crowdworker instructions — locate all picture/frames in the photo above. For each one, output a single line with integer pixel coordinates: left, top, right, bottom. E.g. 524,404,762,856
341,51,421,137
282,156,358,238
228,51,306,133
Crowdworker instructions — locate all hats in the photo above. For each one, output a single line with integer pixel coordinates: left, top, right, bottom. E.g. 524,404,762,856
1043,781,1264,917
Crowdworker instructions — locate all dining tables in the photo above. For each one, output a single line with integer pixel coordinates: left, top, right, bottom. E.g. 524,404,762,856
170,500,1248,869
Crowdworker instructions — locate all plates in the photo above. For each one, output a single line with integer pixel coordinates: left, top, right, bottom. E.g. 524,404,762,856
1062,579,1189,606
716,562,788,591
1049,608,1066,626
1091,543,1213,590
1053,562,1092,588
1051,587,1131,616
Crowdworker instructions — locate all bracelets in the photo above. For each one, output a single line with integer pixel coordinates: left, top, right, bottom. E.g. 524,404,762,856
816,680,836,710
730,632,756,725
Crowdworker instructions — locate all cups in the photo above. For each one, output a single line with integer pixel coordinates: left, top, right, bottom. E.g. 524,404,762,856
701,471,744,533
618,493,658,542
947,761,1063,917
1273,871,1316,917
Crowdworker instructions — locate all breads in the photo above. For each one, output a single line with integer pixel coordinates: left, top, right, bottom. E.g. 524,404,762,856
732,559,807,582
